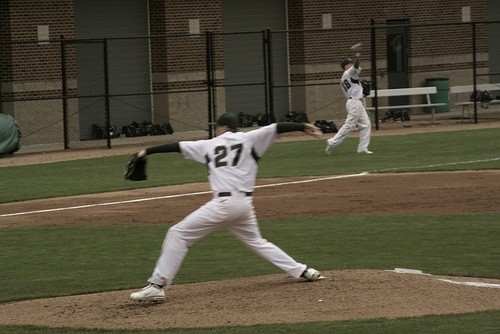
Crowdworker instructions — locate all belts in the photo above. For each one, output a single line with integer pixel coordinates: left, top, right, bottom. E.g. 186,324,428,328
348,97,362,100
218,191,252,196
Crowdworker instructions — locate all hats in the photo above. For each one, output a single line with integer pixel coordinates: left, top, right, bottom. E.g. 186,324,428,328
216,113,239,128
341,58,354,69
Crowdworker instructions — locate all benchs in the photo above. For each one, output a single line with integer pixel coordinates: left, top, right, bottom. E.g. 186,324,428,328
450,82,500,123
364,86,447,128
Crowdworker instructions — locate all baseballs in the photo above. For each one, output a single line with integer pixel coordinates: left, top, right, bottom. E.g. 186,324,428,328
314,130,323,139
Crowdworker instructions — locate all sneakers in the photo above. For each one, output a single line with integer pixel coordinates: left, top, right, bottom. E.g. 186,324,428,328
357,149,374,154
304,267,321,281
129,284,166,300
325,139,332,155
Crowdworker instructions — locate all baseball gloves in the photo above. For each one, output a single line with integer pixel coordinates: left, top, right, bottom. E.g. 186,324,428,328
359,79,371,96
123,152,146,181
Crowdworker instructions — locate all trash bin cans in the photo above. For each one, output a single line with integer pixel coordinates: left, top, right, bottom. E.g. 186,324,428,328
425,78,449,113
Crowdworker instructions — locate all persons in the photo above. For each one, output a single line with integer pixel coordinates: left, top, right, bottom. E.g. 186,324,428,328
326,52,373,154
130,112,322,301
